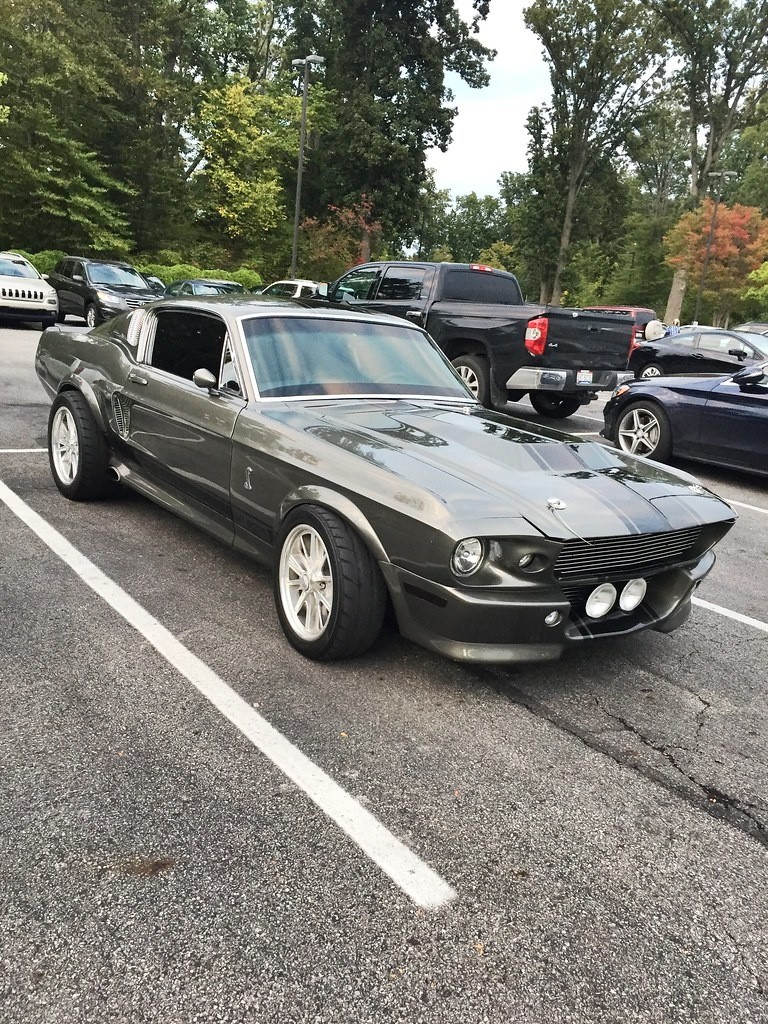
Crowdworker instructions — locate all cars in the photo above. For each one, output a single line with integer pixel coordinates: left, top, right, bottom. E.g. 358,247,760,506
676,320,768,337
142,271,320,301
628,328,768,379
601,352,768,479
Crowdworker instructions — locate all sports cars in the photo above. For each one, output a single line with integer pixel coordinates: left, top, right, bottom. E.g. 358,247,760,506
33,298,736,664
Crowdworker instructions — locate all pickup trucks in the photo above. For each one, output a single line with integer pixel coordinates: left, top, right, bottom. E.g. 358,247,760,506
309,260,638,420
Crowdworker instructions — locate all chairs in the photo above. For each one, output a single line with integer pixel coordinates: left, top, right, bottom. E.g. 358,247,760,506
725,338,740,355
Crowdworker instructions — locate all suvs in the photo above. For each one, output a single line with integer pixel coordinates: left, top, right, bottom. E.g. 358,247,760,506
48,255,165,328
0,251,58,331
577,305,666,356
